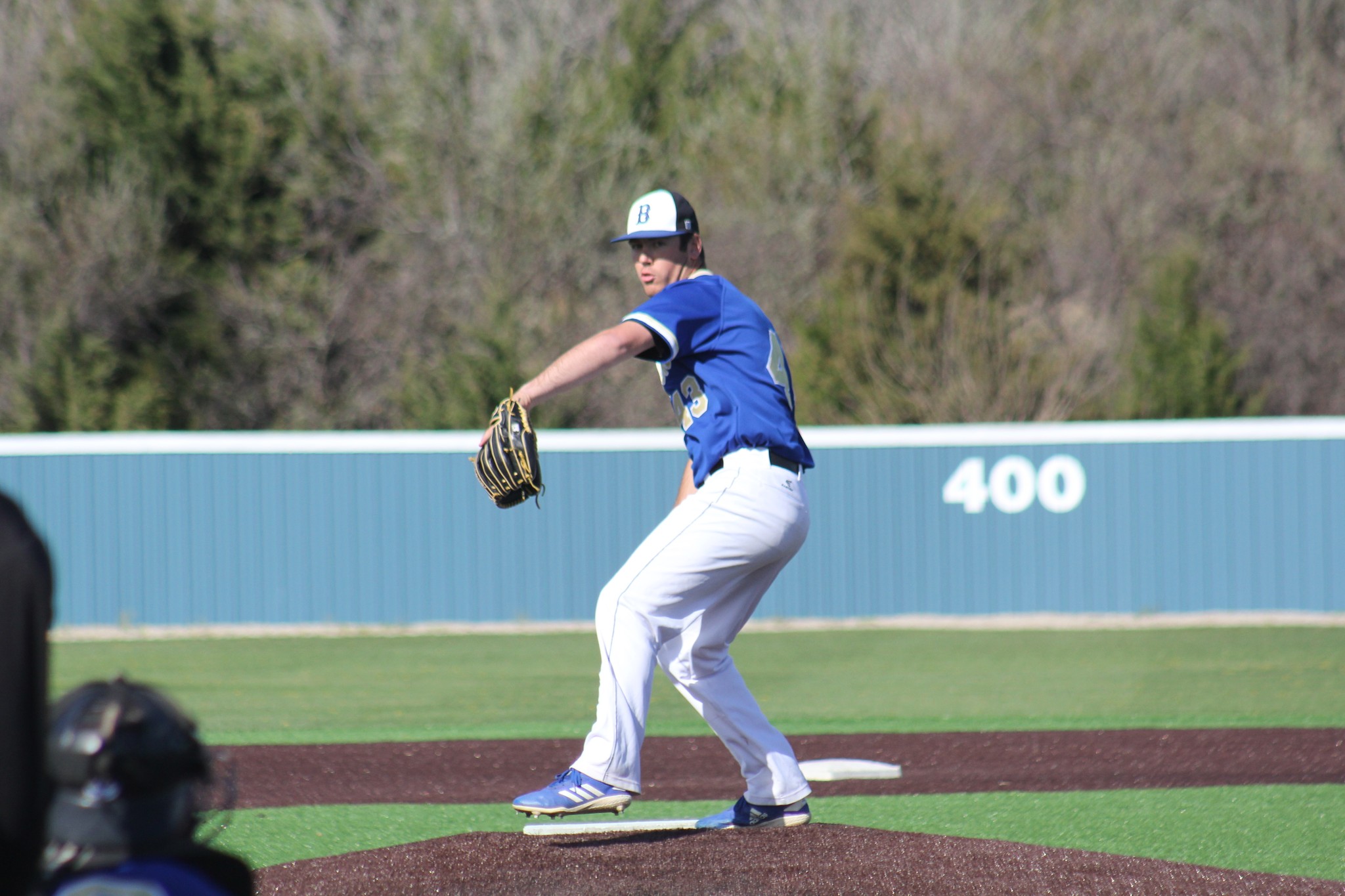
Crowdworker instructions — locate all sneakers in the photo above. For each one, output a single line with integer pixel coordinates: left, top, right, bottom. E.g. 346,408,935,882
695,795,811,829
512,768,632,820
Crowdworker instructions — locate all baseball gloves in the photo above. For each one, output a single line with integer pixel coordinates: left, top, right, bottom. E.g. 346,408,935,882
473,397,542,510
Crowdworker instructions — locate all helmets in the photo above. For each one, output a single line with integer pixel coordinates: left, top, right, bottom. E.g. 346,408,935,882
39,677,216,783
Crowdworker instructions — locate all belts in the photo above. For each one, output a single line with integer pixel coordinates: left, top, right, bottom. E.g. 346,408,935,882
698,451,804,489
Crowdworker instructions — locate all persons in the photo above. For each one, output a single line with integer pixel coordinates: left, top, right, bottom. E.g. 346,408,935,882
42,673,257,896
479,188,815,829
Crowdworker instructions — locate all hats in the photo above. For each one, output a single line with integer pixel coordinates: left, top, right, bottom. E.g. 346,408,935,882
610,189,699,243
